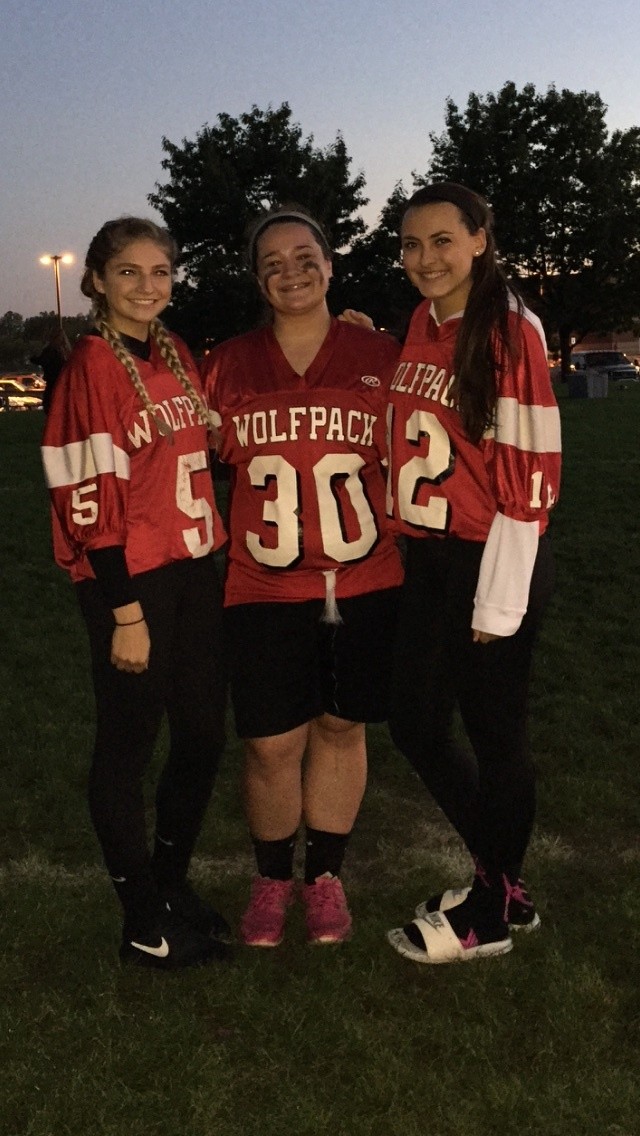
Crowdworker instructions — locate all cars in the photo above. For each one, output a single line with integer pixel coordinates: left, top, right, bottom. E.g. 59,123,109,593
2,374,46,389
0,379,44,404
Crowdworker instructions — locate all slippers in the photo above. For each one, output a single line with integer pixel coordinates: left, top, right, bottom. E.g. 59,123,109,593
416,886,542,935
387,910,515,964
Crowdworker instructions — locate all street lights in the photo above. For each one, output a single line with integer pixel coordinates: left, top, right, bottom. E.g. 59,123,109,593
40,255,73,330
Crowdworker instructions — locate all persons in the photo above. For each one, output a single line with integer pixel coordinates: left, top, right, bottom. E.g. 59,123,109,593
39,212,238,979
202,213,400,951
337,183,564,960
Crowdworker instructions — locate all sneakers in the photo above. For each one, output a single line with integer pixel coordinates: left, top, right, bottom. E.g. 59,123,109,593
239,870,297,946
159,883,231,958
120,919,231,972
299,875,353,946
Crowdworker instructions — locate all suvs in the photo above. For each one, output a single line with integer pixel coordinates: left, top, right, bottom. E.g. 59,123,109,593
570,349,635,387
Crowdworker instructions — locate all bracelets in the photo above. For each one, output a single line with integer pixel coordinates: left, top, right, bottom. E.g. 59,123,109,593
114,616,143,625
